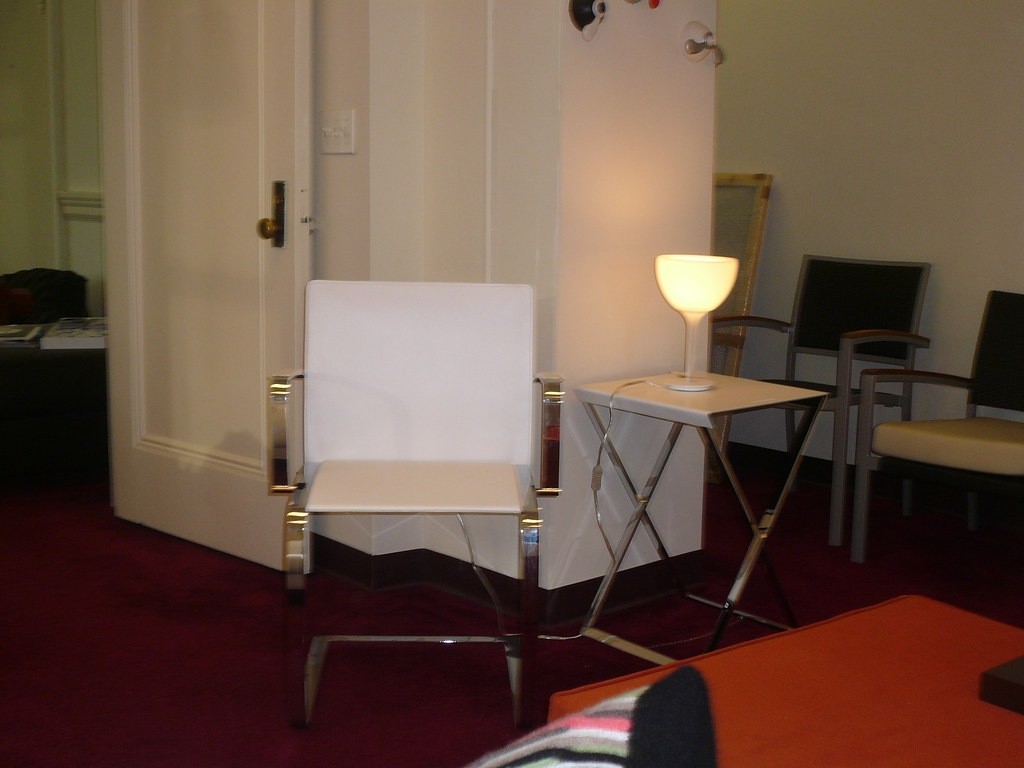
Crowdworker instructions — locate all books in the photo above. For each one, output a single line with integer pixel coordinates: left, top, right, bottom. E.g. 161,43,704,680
40,317,105,348
0,325,45,347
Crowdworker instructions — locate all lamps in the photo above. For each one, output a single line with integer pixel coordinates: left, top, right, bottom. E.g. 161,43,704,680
654,255,742,393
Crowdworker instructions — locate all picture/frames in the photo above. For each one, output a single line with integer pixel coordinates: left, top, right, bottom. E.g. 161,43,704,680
705,171,772,486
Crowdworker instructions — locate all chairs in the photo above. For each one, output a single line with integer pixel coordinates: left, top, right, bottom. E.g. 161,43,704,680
264,277,563,736
0,268,90,326
710,254,931,553
849,290,1024,566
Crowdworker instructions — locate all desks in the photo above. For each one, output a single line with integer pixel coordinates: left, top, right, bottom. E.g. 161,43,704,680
547,593,1024,768
577,370,831,664
0,347,109,497
